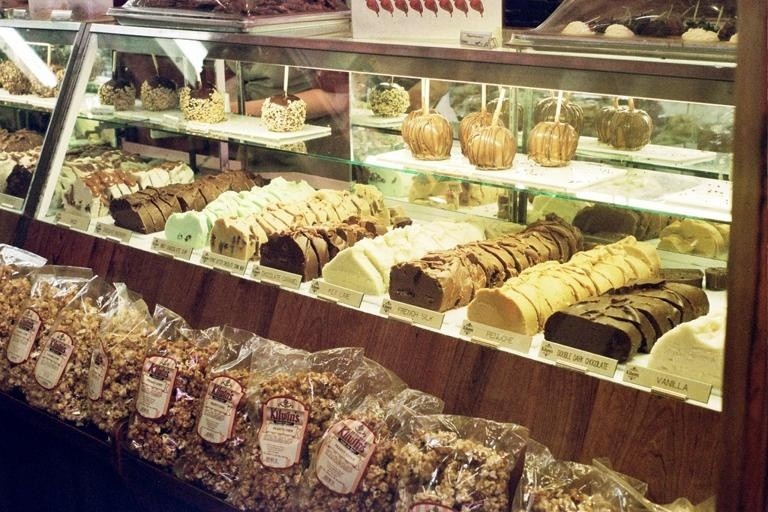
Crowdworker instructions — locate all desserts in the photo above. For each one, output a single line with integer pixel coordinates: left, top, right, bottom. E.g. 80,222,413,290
0,55,581,171
0,125,726,392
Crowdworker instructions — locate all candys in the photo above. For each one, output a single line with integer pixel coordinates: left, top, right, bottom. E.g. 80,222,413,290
580,97,653,150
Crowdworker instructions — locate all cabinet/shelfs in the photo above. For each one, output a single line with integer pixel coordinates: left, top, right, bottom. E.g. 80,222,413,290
20,1,767,512
0,17,115,248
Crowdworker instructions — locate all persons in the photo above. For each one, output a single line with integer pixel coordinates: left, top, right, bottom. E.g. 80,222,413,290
221,57,352,184
112,49,230,163
